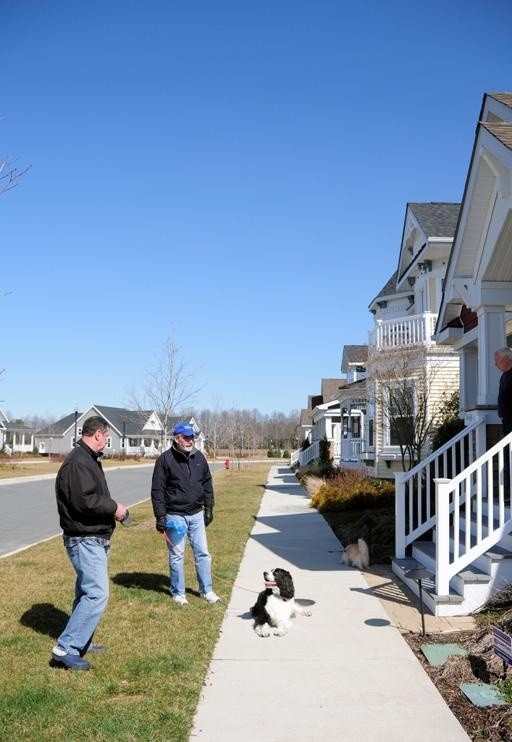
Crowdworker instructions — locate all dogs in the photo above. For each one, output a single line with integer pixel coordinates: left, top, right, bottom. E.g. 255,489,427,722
250,567,313,638
338,537,371,572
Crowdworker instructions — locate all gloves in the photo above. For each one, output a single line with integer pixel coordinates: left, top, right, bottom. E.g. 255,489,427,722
203,508,213,527
120,510,139,529
156,516,166,534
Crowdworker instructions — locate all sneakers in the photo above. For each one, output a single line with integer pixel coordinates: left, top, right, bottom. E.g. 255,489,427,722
172,594,190,606
52,651,91,671
199,591,221,604
89,646,106,653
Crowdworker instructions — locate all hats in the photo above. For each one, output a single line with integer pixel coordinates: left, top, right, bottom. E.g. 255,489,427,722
172,421,199,438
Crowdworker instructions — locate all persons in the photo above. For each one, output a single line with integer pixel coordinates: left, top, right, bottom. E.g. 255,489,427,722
151,422,221,606
494,348,512,505
51,416,129,671
